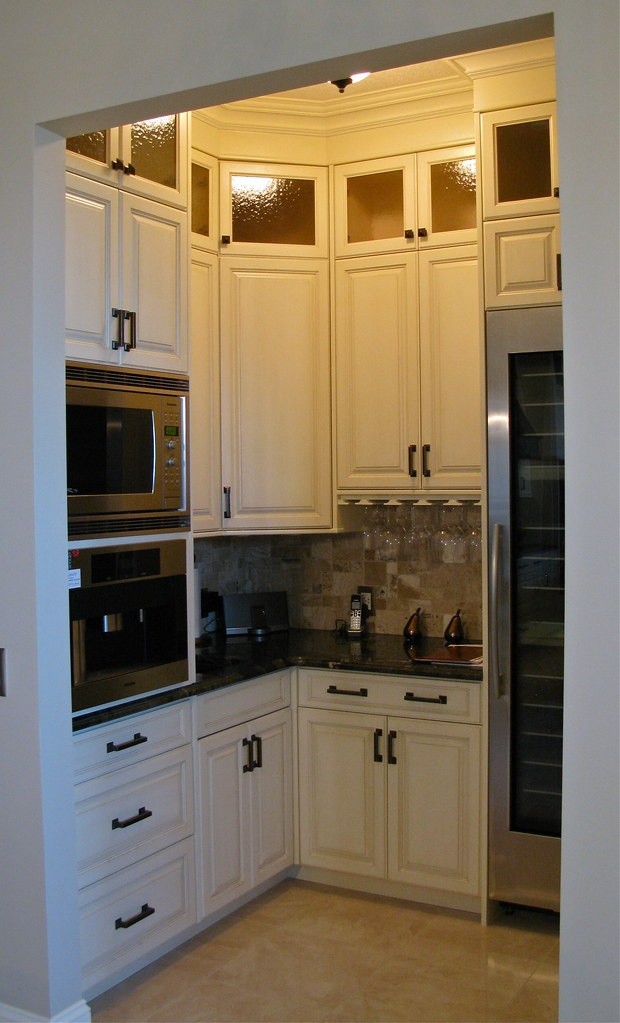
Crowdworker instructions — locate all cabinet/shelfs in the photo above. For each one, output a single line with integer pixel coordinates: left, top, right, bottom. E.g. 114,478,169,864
288,669,482,913
66,111,192,375
480,101,563,310
220,159,333,531
333,142,482,490
73,698,196,1002
191,669,294,937
189,146,220,533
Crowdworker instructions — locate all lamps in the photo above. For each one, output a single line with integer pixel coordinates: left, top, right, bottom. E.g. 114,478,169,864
328,73,371,93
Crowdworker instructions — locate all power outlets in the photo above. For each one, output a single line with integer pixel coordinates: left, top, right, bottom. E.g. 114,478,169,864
357,585,375,617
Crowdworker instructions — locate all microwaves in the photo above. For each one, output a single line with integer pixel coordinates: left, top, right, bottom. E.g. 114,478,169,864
66,359,192,541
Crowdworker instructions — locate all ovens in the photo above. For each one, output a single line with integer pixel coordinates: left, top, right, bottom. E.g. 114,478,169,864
67,539,189,714
